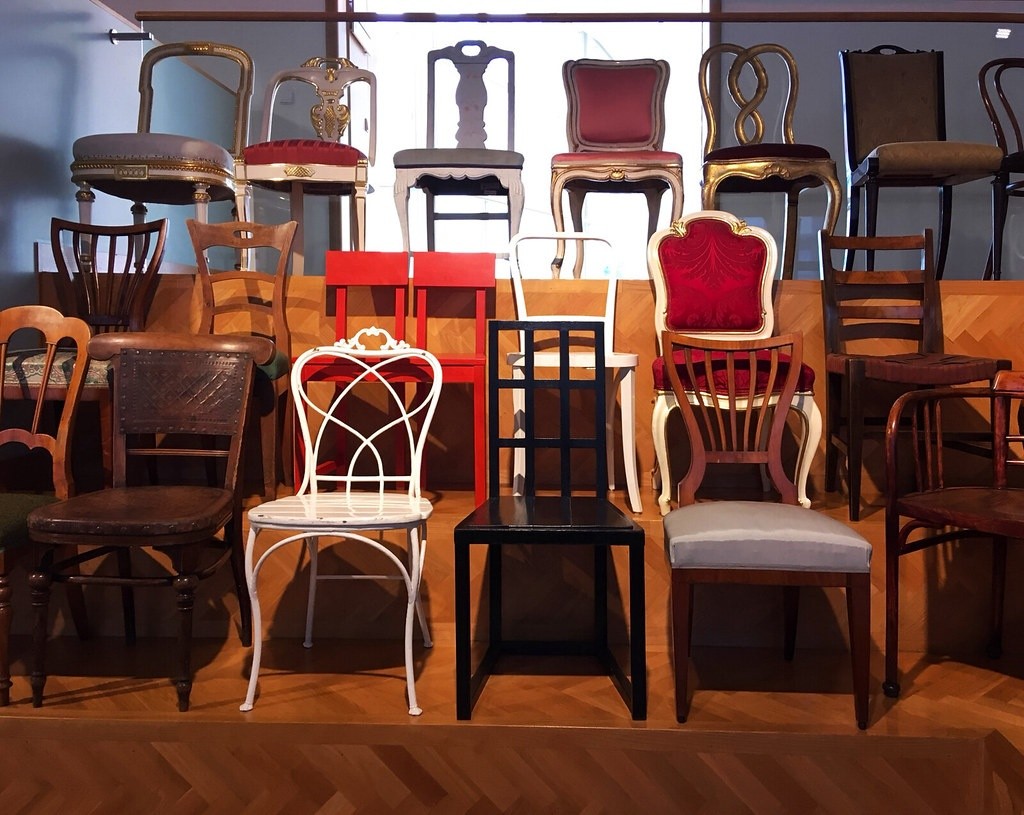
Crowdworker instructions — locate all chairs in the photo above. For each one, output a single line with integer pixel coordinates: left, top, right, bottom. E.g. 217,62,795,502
0,39,1024,731
